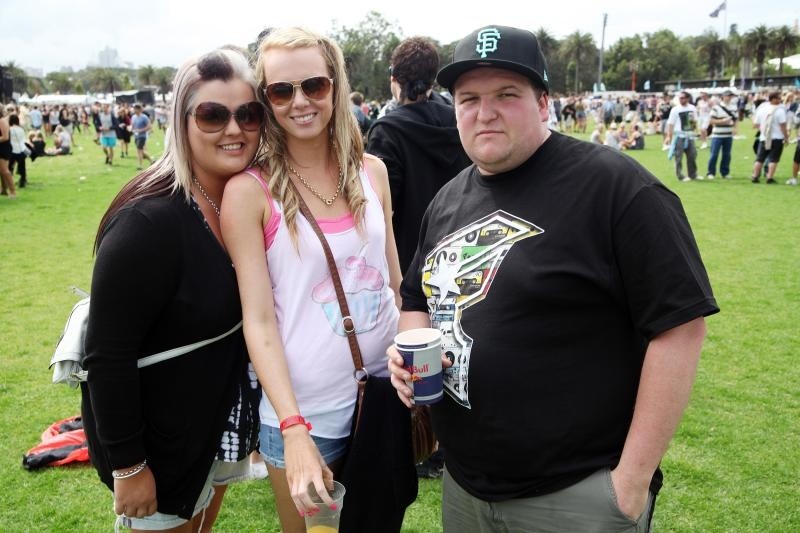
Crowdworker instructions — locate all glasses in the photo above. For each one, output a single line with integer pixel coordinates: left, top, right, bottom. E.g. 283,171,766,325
187,102,266,132
262,76,334,105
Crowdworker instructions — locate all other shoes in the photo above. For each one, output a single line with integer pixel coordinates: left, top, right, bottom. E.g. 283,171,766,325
695,176,704,180
708,175,715,179
250,462,268,479
724,174,732,179
415,462,443,479
766,178,776,184
752,177,760,183
683,177,691,181
786,179,797,184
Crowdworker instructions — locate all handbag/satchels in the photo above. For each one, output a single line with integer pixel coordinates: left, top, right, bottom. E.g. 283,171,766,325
48,288,90,390
350,373,436,458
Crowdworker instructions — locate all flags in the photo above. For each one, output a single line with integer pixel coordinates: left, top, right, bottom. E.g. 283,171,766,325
710,2,726,17
544,70,800,91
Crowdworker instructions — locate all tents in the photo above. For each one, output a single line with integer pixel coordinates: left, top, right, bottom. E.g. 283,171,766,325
12,87,155,106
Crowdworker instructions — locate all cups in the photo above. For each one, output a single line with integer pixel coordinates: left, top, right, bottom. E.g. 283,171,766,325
304,479,346,533
394,327,443,405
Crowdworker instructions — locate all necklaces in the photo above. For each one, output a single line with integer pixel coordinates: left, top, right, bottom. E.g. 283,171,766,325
192,177,220,219
287,161,342,204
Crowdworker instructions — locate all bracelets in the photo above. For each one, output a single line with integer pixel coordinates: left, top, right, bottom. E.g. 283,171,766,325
280,415,312,431
112,459,146,478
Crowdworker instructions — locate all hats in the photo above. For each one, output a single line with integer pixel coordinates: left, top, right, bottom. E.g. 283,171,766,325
436,25,549,97
723,90,734,96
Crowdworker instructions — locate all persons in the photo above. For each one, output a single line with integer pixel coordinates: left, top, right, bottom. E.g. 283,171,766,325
220,32,407,533
364,38,473,481
386,25,720,533
82,50,266,533
0,83,800,196
